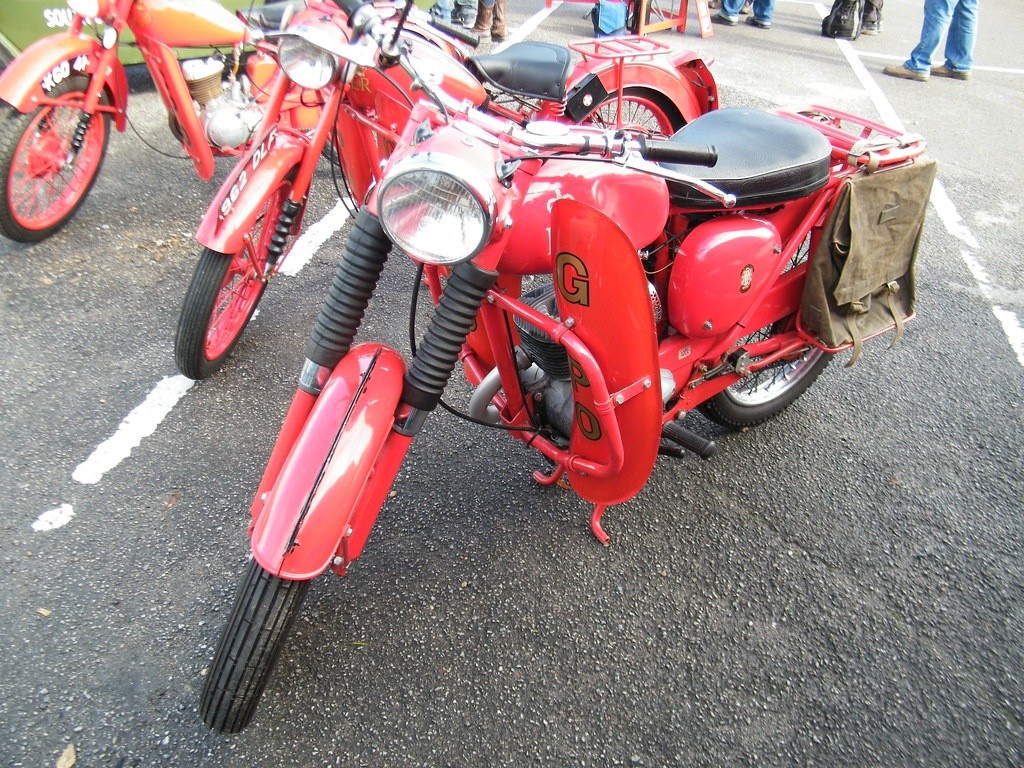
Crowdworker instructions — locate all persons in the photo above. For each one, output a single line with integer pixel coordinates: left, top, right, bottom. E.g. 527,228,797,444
707,0,754,15
471,0,509,42
710,0,775,29
883,0,977,82
861,0,883,36
429,0,478,29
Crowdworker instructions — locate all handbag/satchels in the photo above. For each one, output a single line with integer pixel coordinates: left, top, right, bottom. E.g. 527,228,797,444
806,158,938,367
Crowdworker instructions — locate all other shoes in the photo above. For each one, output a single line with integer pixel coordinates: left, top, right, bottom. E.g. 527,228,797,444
883,64,930,81
710,14,738,25
861,21,882,36
449,8,477,28
746,16,771,29
739,0,754,14
708,0,722,9
930,63,970,80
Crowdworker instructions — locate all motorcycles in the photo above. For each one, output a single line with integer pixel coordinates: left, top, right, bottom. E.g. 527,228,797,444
0,0,328,245
171,0,719,382
196,0,935,736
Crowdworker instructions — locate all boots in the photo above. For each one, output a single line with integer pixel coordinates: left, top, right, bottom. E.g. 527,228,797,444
471,0,496,43
491,0,507,40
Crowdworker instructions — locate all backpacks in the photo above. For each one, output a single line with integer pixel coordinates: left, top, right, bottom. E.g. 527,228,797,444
822,0,864,41
